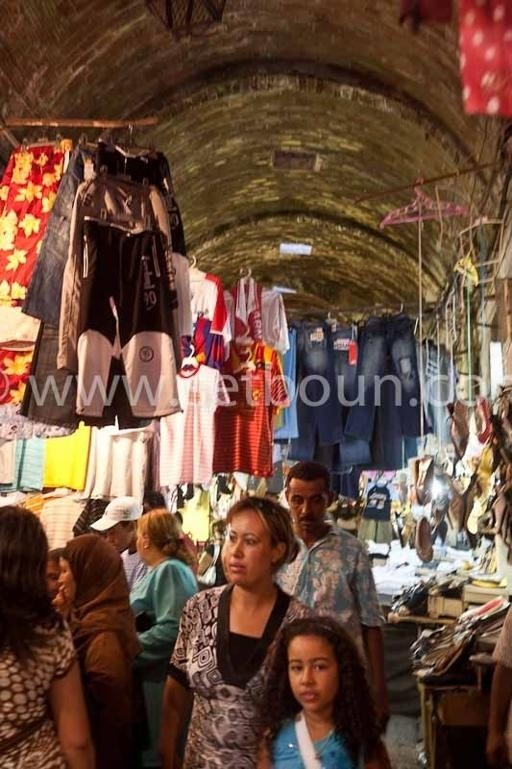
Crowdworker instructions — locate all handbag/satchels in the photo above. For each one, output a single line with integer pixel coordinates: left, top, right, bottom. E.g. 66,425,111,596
196,543,220,586
135,611,153,633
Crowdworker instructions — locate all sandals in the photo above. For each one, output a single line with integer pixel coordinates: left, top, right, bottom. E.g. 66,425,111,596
413,384,512,562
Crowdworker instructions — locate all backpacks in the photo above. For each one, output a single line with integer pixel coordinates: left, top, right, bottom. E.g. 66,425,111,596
391,577,434,616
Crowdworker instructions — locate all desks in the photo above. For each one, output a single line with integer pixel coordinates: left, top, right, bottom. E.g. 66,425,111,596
416,625,491,769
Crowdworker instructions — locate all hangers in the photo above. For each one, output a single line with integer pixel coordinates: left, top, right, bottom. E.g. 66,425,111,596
20,118,512,373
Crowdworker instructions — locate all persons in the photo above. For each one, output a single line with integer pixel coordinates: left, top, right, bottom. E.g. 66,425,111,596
485,603,512,769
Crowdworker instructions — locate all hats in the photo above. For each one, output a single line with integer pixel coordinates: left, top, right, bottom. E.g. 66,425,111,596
90,496,143,531
